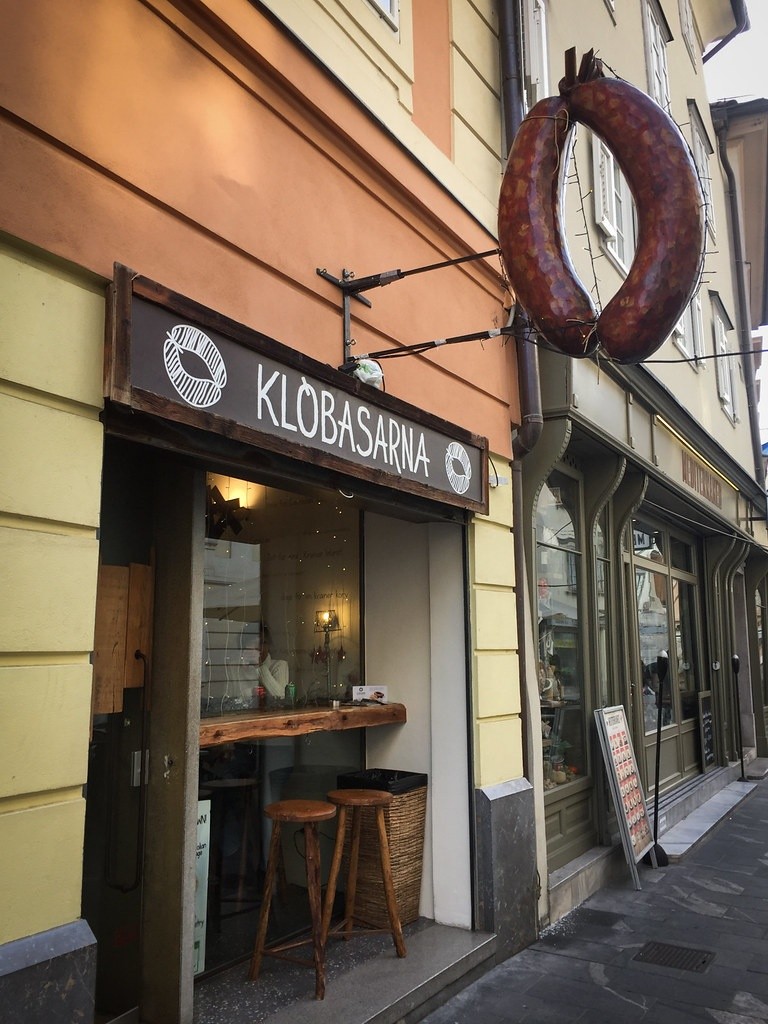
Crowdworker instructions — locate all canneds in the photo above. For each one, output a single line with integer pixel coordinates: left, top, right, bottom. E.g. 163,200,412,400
252,685,264,710
285,684,296,705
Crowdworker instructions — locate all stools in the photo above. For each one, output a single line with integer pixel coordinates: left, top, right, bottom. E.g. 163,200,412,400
320,790,407,958
249,799,335,1001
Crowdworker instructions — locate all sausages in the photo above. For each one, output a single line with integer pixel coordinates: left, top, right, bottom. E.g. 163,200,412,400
497,96,601,358
559,74,704,365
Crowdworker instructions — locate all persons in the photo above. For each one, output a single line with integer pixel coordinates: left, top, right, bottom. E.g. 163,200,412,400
223,622,294,805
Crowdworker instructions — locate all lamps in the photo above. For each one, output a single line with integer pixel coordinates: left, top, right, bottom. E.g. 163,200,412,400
316,609,338,702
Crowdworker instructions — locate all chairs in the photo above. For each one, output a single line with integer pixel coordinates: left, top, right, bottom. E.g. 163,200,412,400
202,778,278,937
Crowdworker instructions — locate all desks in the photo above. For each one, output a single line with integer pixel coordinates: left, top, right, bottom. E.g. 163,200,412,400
198,703,406,748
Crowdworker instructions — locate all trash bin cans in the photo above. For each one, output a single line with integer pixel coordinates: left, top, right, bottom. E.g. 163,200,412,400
334,767,429,931
